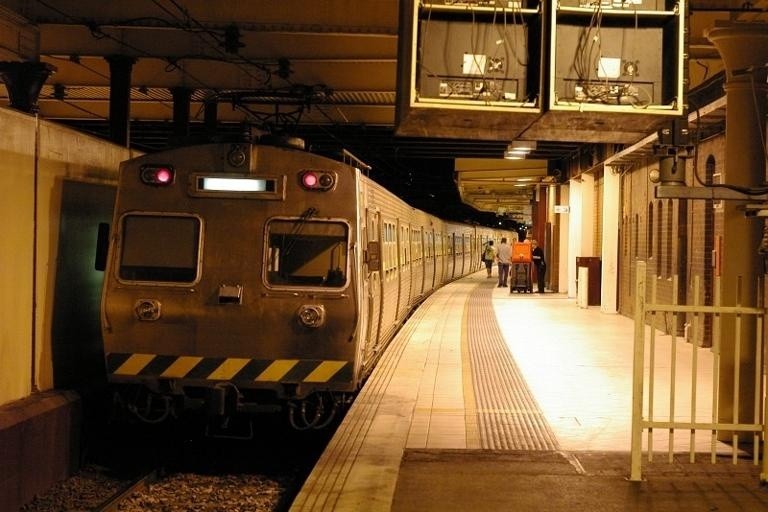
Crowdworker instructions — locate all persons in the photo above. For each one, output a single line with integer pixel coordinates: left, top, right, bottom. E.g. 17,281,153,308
531,239,546,293
496,238,511,287
483,240,496,278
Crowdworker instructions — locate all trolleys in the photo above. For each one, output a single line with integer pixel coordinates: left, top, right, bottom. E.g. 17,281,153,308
506,260,535,293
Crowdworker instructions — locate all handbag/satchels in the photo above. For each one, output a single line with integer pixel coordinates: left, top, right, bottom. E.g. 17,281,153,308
481,251,486,262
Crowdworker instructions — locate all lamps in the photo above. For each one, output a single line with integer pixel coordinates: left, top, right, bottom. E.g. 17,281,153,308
504,139,538,163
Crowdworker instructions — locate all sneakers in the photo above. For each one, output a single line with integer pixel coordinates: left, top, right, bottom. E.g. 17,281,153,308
535,289,544,293
503,284,508,287
497,283,502,288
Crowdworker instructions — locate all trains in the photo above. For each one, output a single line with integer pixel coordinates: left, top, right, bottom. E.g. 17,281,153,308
80,131,518,439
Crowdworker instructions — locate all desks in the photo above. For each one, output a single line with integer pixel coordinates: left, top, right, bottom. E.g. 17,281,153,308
576,256,601,306
